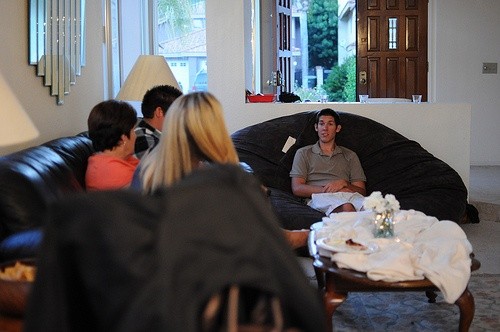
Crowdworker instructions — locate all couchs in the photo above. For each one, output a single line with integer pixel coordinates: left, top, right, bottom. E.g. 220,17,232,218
229,110,480,225
0,128,95,265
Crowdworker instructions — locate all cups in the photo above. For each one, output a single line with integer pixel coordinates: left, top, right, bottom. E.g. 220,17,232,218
359,94,369,102
320,95,328,102
412,95,421,102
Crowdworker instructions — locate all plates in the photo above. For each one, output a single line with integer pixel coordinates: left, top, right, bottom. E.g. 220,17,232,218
316,237,379,254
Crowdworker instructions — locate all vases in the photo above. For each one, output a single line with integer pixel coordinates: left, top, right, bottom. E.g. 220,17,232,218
370,212,395,239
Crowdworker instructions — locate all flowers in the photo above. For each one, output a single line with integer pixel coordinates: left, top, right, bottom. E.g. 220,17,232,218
361,190,401,225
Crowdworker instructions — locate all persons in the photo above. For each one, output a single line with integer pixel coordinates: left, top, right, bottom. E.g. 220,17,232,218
289,108,367,215
85,99,140,188
131,91,240,192
132,85,310,250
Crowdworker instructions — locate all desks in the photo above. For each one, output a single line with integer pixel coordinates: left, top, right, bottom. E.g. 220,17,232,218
304,208,482,332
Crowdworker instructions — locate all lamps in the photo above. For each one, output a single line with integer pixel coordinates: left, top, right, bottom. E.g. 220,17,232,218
115,54,181,101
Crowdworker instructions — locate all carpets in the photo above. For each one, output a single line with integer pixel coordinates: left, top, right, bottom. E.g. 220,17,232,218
306,271,500,332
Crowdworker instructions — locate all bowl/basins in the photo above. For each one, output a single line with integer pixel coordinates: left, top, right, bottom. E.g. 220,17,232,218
248,94,277,102
0,258,38,315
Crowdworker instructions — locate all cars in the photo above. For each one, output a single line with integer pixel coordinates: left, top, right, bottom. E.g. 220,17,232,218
191,66,208,93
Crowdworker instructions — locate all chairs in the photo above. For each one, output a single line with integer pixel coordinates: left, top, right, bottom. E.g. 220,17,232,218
151,159,331,332
19,183,163,332
